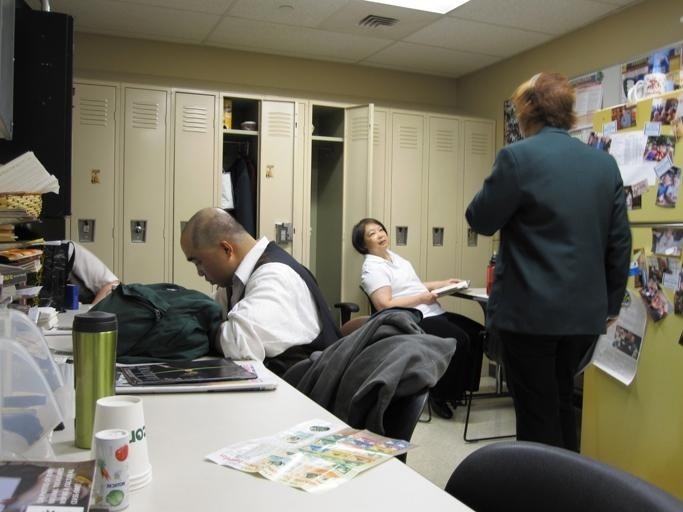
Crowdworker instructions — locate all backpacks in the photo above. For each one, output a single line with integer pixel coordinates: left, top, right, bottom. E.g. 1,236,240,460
88,283,221,362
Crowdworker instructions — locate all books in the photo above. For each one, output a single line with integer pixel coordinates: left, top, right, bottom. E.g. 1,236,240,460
1,210,43,309
113,357,278,395
431,280,470,298
180,208,342,379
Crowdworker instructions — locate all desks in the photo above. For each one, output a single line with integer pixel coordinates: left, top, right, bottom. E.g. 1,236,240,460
0,305,474,512
451,289,517,442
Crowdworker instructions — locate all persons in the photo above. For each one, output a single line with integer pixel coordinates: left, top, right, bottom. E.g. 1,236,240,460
12,222,118,304
353,219,482,421
465,72,632,451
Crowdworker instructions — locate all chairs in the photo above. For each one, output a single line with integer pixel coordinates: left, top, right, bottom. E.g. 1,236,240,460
281,359,429,463
359,284,467,423
334,303,360,326
444,442,683,512
338,316,369,337
15,219,66,241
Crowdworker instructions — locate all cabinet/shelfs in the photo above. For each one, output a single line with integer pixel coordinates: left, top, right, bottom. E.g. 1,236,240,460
217,94,299,261
423,113,462,282
168,89,220,299
306,101,372,329
299,99,308,267
458,116,496,378
373,108,389,229
66,79,120,277
13,9,73,216
389,110,426,280
120,84,170,284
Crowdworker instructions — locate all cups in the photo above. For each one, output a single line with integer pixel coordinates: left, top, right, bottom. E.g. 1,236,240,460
92,428,130,511
71,310,118,450
90,394,151,492
66,284,80,310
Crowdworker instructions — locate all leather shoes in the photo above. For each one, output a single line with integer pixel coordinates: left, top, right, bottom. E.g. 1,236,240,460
431,391,454,420
